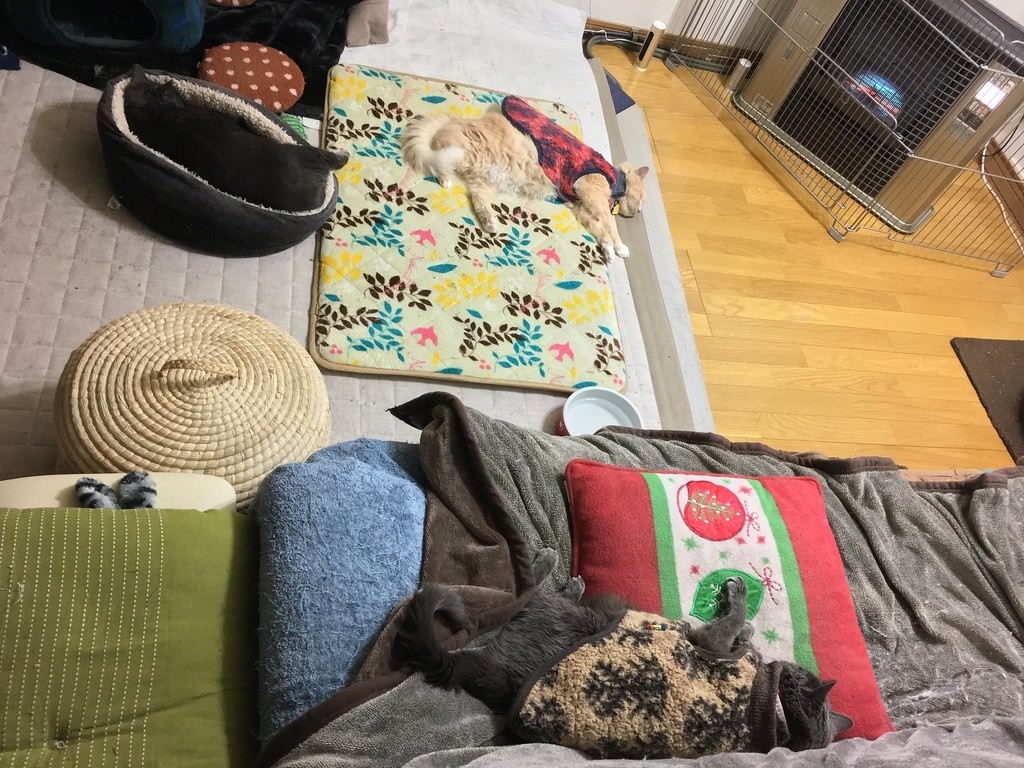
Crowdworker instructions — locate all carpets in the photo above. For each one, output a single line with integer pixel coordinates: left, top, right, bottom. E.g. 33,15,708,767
951,337,1024,468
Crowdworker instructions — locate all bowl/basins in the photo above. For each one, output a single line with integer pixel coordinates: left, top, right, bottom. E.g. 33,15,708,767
555,386,645,437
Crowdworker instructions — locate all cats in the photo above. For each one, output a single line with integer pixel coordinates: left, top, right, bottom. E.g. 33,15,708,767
396,547,854,758
396,112,650,268
124,63,349,212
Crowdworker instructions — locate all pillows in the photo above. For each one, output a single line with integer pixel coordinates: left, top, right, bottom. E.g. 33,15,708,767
565,458,897,745
